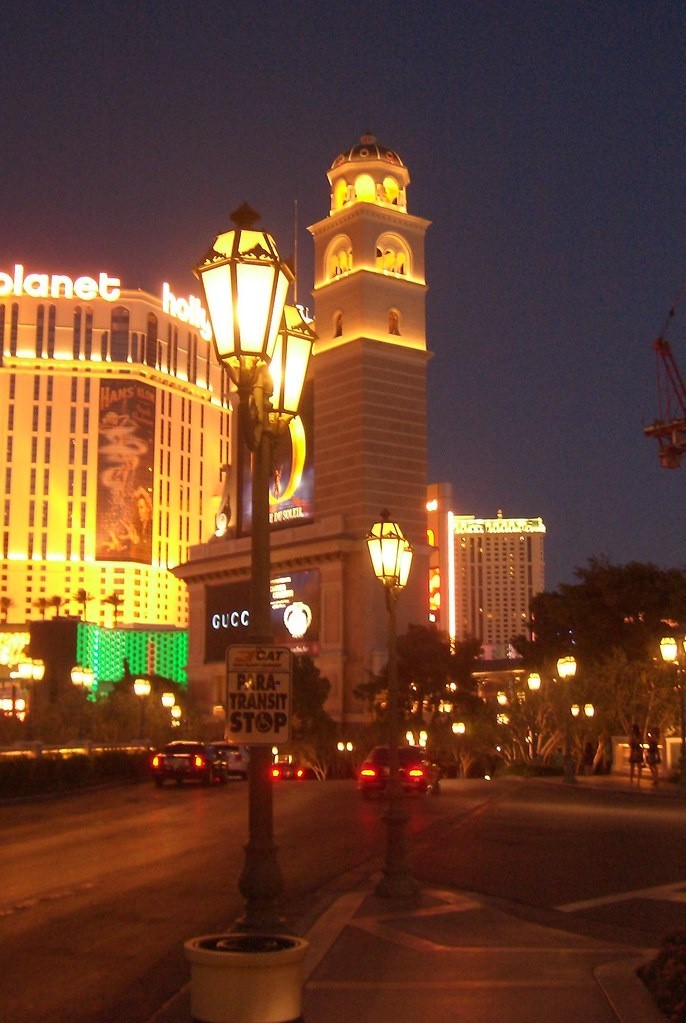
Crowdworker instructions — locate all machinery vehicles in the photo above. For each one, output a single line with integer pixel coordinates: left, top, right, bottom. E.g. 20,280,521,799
639,277,686,470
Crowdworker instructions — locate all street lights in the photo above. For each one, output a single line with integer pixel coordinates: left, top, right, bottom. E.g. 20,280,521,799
527,672,543,778
659,634,686,804
450,722,465,767
555,654,577,784
69,666,94,741
17,658,46,742
186,203,317,933
571,702,596,753
133,678,152,738
364,506,423,903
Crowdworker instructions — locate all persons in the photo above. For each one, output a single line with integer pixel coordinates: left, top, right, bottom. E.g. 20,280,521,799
273,466,284,500
552,724,668,787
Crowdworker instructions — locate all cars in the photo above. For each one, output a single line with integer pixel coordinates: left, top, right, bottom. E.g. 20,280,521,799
356,744,442,799
149,738,229,788
210,738,251,780
